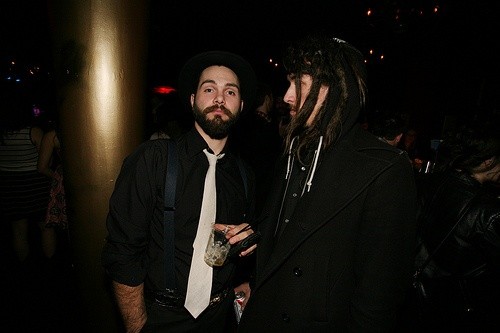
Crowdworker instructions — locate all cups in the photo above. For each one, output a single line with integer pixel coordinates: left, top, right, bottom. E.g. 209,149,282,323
203,222,233,267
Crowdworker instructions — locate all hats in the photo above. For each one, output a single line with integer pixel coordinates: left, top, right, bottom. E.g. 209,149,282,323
177,50,260,108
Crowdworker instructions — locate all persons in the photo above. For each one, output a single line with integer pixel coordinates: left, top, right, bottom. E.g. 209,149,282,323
104,42,500,308
0,95,75,269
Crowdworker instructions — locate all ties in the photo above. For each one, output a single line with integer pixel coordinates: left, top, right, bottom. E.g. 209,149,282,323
184,150,225,320
